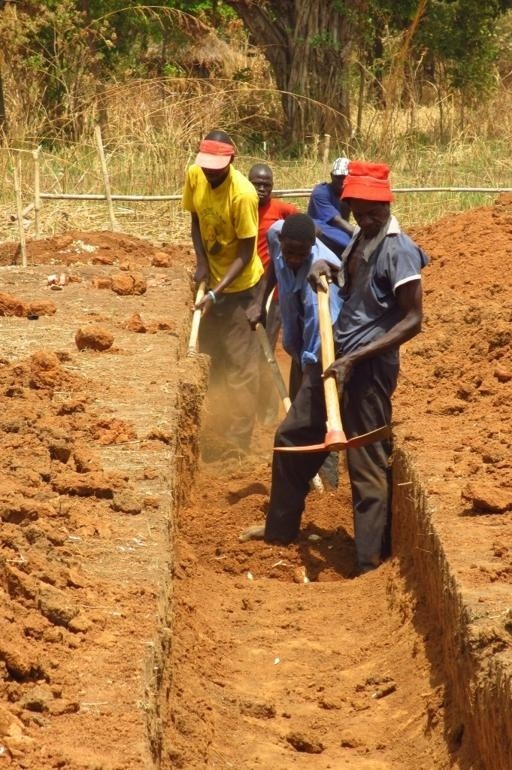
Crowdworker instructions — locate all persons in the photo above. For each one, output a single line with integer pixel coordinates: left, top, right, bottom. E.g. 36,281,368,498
183,132,266,462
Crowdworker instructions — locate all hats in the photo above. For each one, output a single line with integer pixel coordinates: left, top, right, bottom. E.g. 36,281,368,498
330,157,395,202
195,140,235,170
